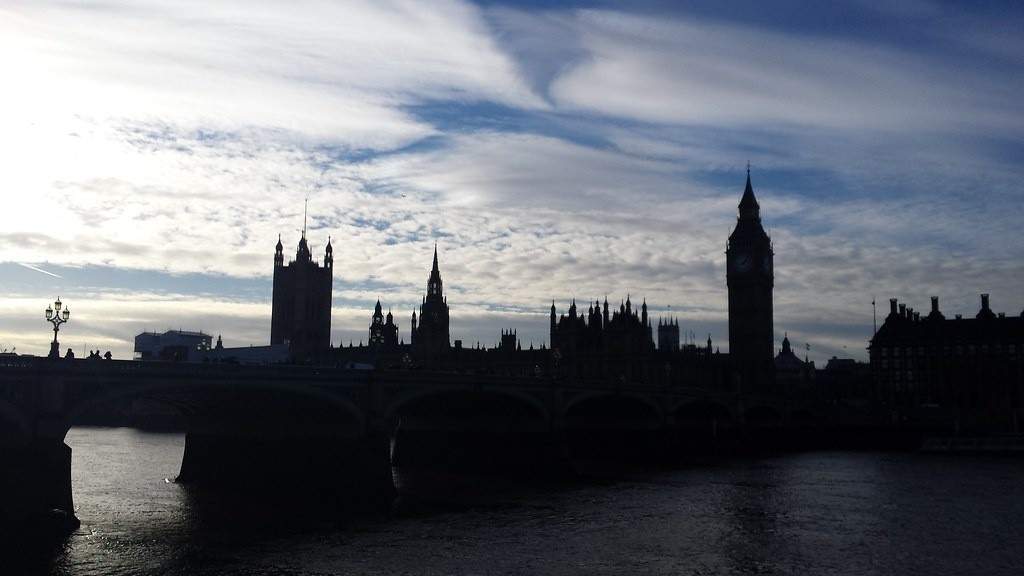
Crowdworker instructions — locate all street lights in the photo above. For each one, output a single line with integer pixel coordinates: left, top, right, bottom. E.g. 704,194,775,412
46,303,70,361
